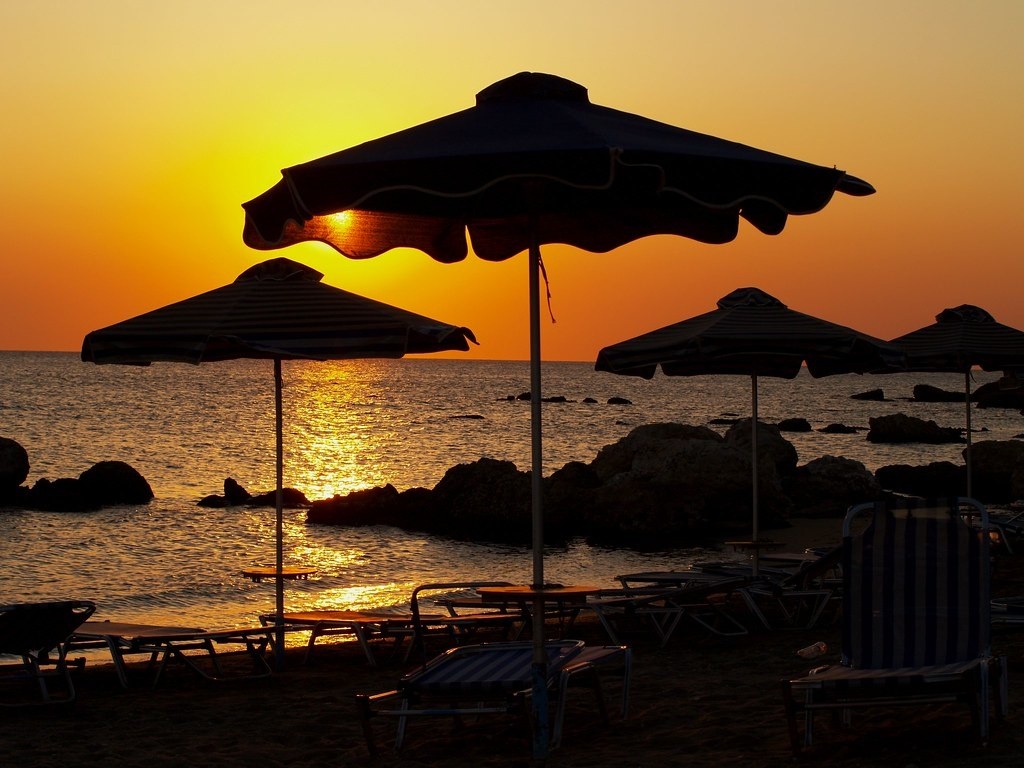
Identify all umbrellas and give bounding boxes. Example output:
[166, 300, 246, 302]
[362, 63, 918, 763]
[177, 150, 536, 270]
[79, 256, 481, 645]
[596, 287, 1024, 578]
[240, 71, 878, 665]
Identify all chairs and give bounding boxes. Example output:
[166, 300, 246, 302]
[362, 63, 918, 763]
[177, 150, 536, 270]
[0, 497, 1024, 762]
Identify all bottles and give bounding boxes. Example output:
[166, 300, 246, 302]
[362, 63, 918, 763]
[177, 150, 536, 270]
[796, 642, 830, 658]
